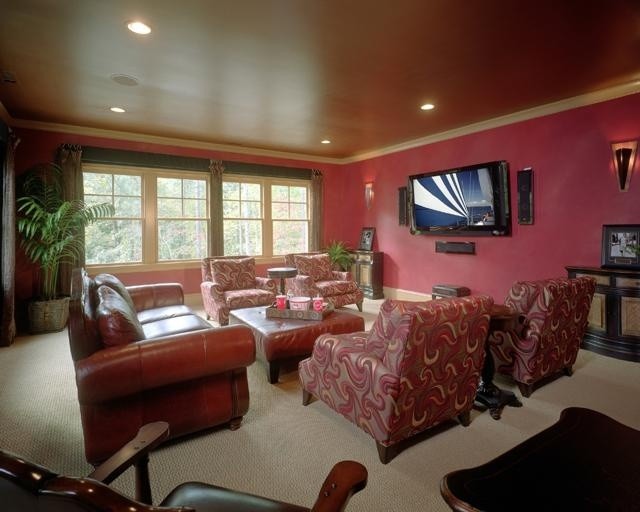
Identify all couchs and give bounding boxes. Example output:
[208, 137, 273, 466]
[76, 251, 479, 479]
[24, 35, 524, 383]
[67, 264, 257, 467]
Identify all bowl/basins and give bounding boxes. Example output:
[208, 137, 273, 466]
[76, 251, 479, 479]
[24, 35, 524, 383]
[289, 296, 312, 312]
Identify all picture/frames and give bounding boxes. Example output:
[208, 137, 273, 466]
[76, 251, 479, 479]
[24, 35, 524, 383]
[601, 221, 638, 271]
[357, 225, 376, 252]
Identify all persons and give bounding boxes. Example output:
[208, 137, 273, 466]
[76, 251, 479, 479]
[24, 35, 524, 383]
[619, 233, 628, 256]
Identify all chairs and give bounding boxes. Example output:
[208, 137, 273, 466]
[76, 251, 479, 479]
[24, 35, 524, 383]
[0, 417, 368, 511]
[200, 251, 364, 326]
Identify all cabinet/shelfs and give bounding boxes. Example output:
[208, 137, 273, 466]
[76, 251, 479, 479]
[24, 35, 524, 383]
[345, 252, 384, 299]
[565, 271, 640, 363]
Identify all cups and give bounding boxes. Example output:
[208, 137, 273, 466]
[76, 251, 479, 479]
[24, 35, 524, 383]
[275, 295, 287, 311]
[313, 297, 325, 312]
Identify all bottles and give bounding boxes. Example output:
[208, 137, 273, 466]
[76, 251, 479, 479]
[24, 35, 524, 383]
[286, 288, 295, 300]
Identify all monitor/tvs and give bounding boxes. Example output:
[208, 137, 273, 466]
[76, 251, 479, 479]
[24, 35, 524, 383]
[409, 160, 512, 238]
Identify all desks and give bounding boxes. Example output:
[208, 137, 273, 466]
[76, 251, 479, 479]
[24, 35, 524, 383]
[432, 404, 640, 512]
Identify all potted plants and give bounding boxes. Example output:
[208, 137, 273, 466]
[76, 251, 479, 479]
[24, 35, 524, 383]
[14, 155, 118, 334]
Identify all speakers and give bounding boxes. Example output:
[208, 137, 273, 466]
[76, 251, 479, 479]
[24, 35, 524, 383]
[516, 168, 534, 225]
[398, 187, 409, 225]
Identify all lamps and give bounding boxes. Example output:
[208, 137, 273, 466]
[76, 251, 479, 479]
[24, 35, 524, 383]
[609, 141, 638, 194]
